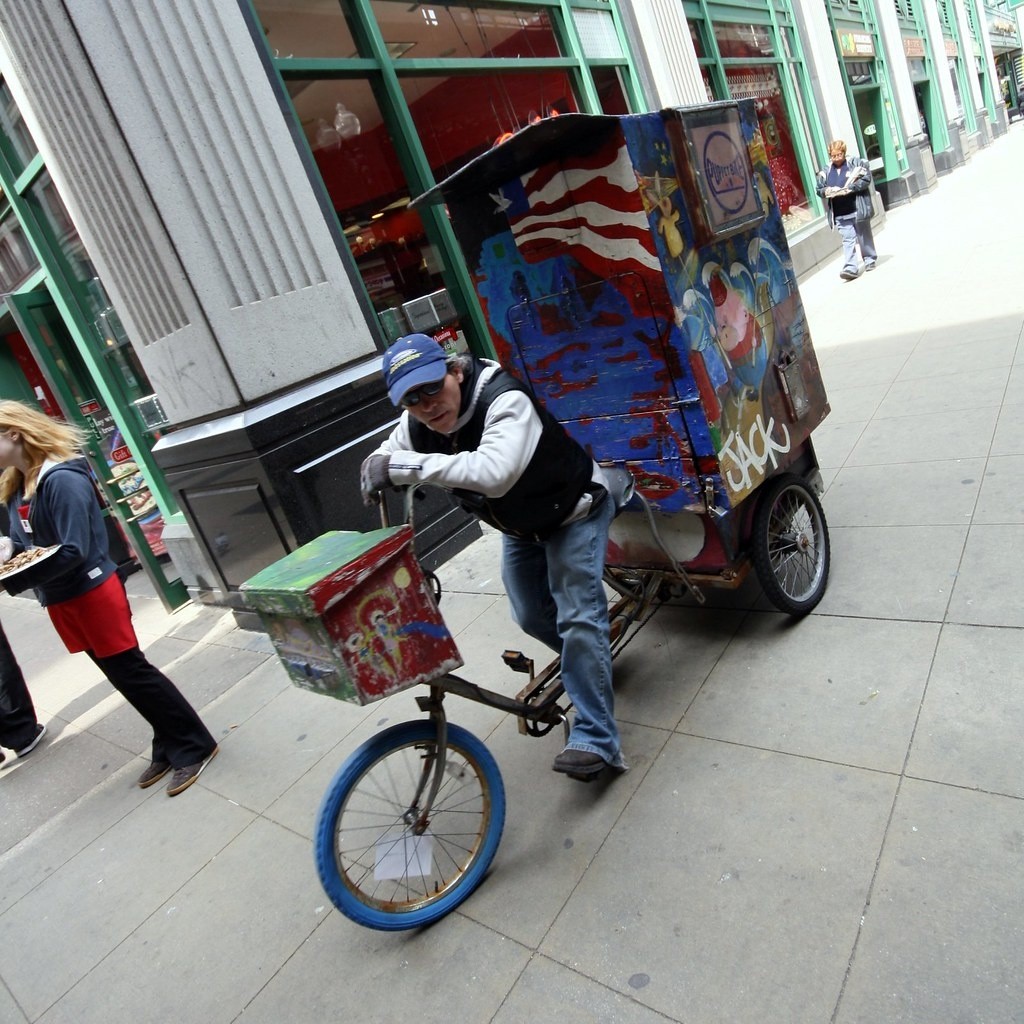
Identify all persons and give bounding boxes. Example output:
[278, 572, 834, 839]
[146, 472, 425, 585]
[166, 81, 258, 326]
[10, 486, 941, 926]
[816, 140, 876, 279]
[360, 333, 629, 782]
[0, 400, 220, 796]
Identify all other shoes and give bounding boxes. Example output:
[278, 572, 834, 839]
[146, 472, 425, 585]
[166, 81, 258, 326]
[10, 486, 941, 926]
[840, 270, 857, 278]
[866, 262, 877, 272]
[14, 723, 47, 756]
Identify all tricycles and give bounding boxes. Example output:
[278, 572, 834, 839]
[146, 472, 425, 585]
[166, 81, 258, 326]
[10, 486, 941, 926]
[237, 93, 832, 933]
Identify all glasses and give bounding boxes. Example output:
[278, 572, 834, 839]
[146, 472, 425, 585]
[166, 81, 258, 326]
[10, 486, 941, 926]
[402, 376, 445, 407]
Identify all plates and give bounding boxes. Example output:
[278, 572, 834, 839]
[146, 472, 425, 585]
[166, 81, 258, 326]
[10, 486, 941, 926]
[0, 545, 60, 580]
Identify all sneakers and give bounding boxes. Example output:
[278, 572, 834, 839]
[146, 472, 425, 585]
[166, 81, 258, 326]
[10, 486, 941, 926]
[138, 758, 174, 788]
[552, 748, 606, 775]
[165, 743, 219, 796]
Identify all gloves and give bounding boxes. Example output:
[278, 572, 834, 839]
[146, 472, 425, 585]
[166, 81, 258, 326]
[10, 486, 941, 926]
[359, 454, 394, 507]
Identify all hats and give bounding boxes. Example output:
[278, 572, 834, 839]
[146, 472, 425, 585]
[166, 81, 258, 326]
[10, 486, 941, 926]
[382, 333, 453, 406]
